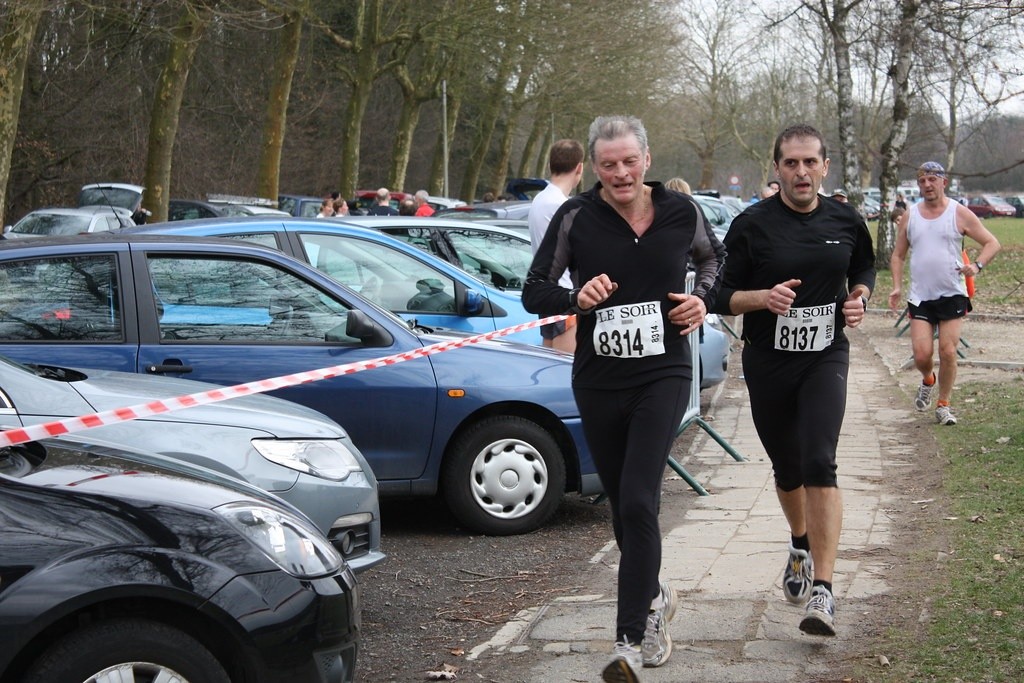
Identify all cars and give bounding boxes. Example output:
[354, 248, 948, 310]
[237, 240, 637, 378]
[0, 182, 745, 392]
[0, 234, 604, 539]
[857, 188, 922, 221]
[14, 214, 549, 348]
[999, 195, 1024, 219]
[0, 425, 364, 683]
[0, 347, 389, 577]
[964, 194, 1017, 220]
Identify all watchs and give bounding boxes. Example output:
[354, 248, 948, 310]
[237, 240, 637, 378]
[974, 261, 983, 272]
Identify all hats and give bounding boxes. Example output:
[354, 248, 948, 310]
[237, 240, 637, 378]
[829, 189, 847, 197]
[917, 161, 944, 179]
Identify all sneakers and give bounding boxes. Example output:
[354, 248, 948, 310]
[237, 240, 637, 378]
[936, 405, 956, 426]
[640, 583, 677, 667]
[602, 634, 642, 683]
[782, 542, 815, 604]
[914, 372, 937, 411]
[800, 584, 836, 638]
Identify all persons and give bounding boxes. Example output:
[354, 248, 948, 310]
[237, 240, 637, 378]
[526, 138, 585, 352]
[708, 124, 878, 630]
[750, 181, 780, 202]
[522, 113, 728, 683]
[889, 161, 1000, 424]
[688, 318, 692, 324]
[895, 193, 906, 210]
[830, 189, 848, 202]
[890, 207, 906, 225]
[316, 187, 436, 218]
[664, 177, 691, 195]
[483, 193, 495, 203]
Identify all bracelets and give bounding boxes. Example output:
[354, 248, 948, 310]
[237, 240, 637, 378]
[861, 296, 868, 312]
